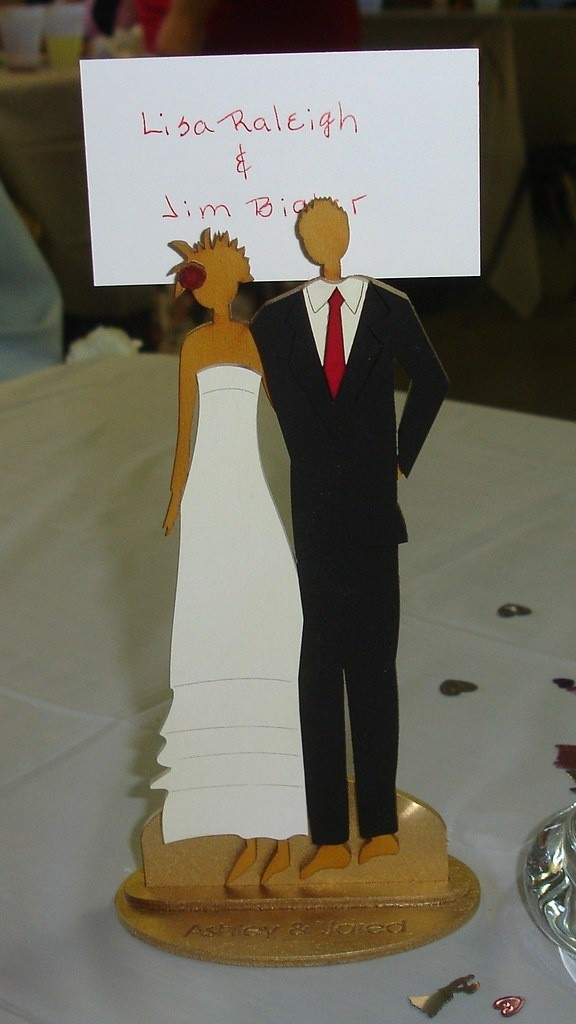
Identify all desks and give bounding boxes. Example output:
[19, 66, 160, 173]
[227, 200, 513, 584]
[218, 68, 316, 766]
[1, 356, 576, 1024]
[357, 8, 575, 318]
[1, 48, 153, 340]
